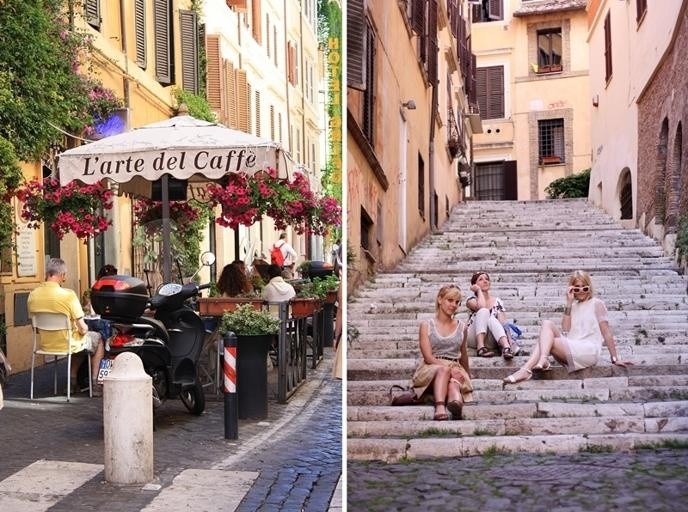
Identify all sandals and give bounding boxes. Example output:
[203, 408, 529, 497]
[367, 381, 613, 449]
[434, 401, 448, 420]
[531, 357, 553, 372]
[502, 367, 533, 384]
[503, 347, 514, 359]
[477, 347, 495, 357]
[448, 401, 463, 420]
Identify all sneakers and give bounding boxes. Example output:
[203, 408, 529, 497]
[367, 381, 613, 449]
[91, 384, 104, 397]
[63, 381, 80, 395]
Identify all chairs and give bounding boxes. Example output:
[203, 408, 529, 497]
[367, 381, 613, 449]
[27, 310, 95, 400]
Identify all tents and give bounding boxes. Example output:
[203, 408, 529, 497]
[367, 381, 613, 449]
[55, 115, 301, 285]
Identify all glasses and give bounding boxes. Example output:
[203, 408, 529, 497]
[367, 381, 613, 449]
[572, 286, 589, 293]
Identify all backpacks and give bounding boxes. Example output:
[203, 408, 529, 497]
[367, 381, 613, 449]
[271, 242, 288, 267]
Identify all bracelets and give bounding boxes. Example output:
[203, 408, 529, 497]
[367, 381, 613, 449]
[565, 307, 571, 315]
[612, 357, 617, 363]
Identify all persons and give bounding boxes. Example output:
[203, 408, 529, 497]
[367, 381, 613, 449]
[413, 284, 473, 420]
[260, 264, 295, 335]
[503, 270, 634, 385]
[462, 271, 515, 359]
[27, 259, 105, 397]
[271, 232, 298, 279]
[217, 264, 253, 298]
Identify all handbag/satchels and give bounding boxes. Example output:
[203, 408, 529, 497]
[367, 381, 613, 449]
[503, 321, 522, 356]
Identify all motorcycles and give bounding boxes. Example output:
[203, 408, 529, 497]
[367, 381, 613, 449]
[89, 250, 216, 429]
